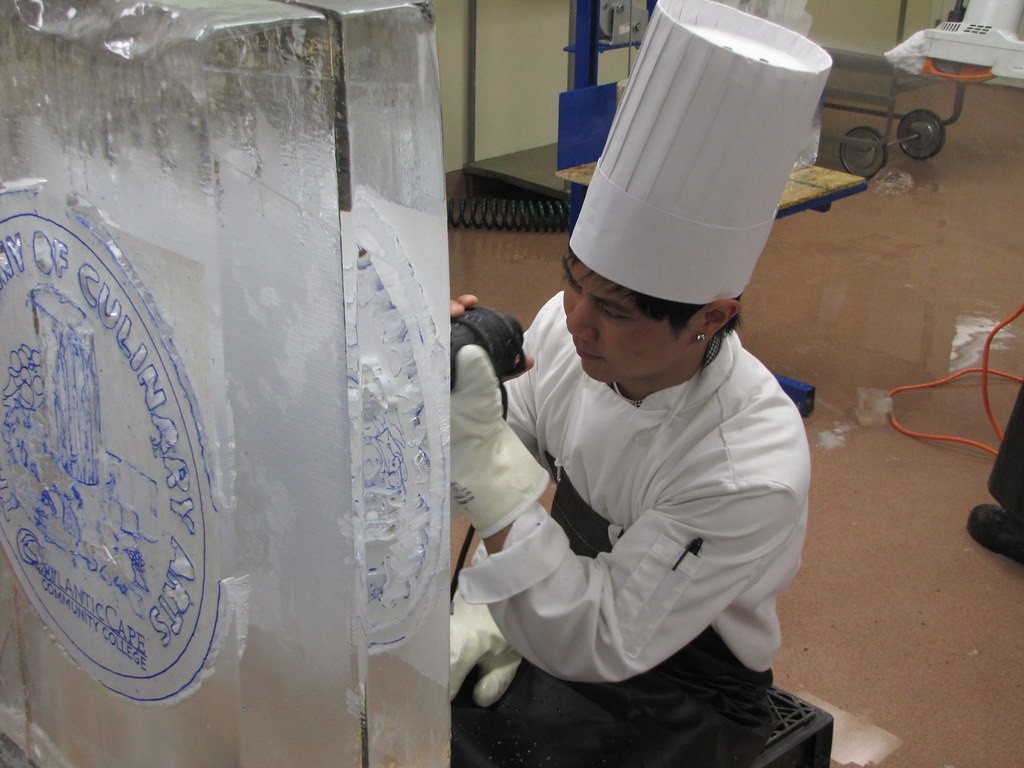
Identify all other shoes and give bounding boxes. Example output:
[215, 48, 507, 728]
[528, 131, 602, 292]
[966, 503, 1024, 564]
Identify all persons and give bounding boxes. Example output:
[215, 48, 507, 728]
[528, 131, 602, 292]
[967, 384, 1024, 565]
[450, 0, 833, 768]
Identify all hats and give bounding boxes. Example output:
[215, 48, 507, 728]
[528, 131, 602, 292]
[568, 0, 834, 306]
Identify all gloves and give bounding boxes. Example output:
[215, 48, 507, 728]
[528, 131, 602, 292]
[449, 343, 550, 539]
